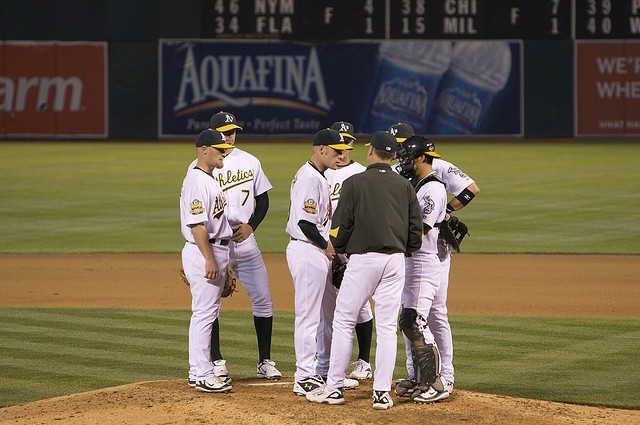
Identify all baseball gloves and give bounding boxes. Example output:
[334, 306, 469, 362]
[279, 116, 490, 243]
[332, 254, 347, 289]
[220, 265, 238, 298]
[440, 216, 468, 253]
[177, 267, 190, 286]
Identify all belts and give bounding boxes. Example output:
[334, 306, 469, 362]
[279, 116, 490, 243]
[209, 238, 229, 245]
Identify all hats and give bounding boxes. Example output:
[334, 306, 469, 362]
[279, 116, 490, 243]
[209, 110, 243, 131]
[365, 130, 396, 150]
[388, 121, 415, 142]
[330, 120, 356, 139]
[196, 129, 235, 148]
[313, 127, 353, 150]
[403, 135, 441, 157]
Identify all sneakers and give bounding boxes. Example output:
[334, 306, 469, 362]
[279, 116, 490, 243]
[343, 377, 359, 388]
[394, 381, 419, 395]
[412, 380, 448, 401]
[187, 378, 198, 386]
[350, 359, 373, 378]
[195, 375, 232, 391]
[213, 359, 228, 376]
[293, 377, 326, 395]
[372, 390, 393, 409]
[305, 385, 345, 403]
[441, 375, 453, 392]
[256, 359, 282, 379]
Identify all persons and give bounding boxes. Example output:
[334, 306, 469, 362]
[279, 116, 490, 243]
[186, 111, 282, 383]
[183, 128, 236, 394]
[306, 129, 421, 410]
[398, 138, 449, 405]
[389, 119, 480, 398]
[319, 121, 374, 381]
[288, 129, 358, 398]
